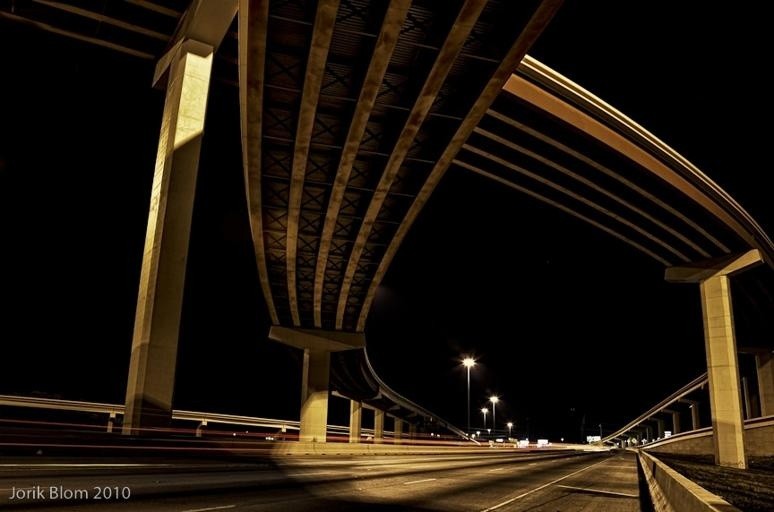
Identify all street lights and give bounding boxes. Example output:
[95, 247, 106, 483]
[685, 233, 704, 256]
[463, 357, 514, 439]
